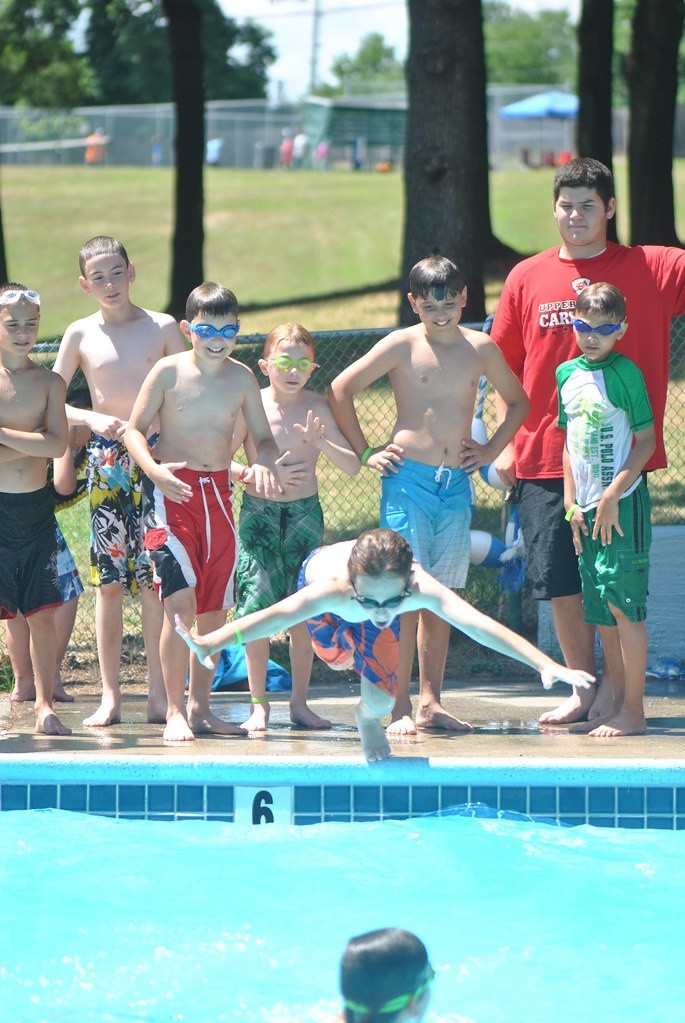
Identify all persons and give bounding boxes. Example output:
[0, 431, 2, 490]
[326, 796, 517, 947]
[124, 282, 284, 741]
[489, 158, 685, 724]
[328, 256, 530, 734]
[205, 130, 222, 167]
[84, 127, 105, 165]
[336, 927, 435, 1023]
[229, 322, 360, 731]
[0, 283, 95, 733]
[177, 528, 597, 763]
[555, 282, 656, 736]
[278, 125, 329, 169]
[53, 236, 186, 727]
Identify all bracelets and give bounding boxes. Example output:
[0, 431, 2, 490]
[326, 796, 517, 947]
[361, 447, 372, 465]
[225, 623, 242, 648]
[565, 504, 578, 521]
[239, 467, 249, 484]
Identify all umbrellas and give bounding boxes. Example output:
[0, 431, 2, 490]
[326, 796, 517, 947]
[499, 88, 580, 166]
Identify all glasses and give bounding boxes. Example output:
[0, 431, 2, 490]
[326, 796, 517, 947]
[570, 316, 628, 336]
[188, 322, 241, 340]
[267, 357, 320, 374]
[349, 578, 413, 610]
[0, 290, 41, 305]
